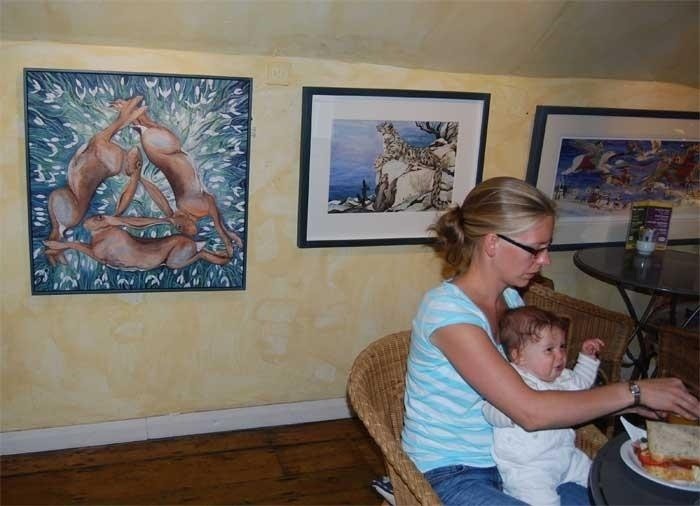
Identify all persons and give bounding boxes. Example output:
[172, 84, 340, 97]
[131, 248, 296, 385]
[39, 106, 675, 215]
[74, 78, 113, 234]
[401, 176, 700, 506]
[482, 308, 606, 506]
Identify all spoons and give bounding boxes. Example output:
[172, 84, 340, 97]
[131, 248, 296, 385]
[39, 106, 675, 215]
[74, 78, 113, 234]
[620, 416, 647, 441]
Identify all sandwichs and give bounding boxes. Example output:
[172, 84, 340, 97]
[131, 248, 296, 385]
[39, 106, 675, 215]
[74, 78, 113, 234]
[631, 420, 700, 481]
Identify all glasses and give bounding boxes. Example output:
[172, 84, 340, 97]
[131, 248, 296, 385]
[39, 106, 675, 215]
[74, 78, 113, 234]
[496, 232, 555, 260]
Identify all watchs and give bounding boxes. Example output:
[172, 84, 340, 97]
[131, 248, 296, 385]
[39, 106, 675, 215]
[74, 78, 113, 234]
[629, 380, 642, 405]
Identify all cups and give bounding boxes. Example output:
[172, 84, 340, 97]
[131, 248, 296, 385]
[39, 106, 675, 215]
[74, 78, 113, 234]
[636, 229, 658, 255]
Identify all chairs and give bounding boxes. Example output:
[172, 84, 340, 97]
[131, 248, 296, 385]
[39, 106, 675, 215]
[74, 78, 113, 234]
[654, 325, 700, 401]
[348, 325, 610, 503]
[520, 274, 639, 383]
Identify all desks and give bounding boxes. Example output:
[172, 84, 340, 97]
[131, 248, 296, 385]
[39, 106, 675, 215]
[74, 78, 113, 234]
[573, 248, 700, 380]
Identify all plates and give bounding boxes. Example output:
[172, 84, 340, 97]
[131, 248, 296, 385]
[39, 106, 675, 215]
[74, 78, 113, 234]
[619, 439, 700, 493]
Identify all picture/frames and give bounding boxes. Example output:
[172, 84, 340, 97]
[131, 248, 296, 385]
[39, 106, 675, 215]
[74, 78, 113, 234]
[298, 82, 493, 251]
[524, 104, 700, 251]
